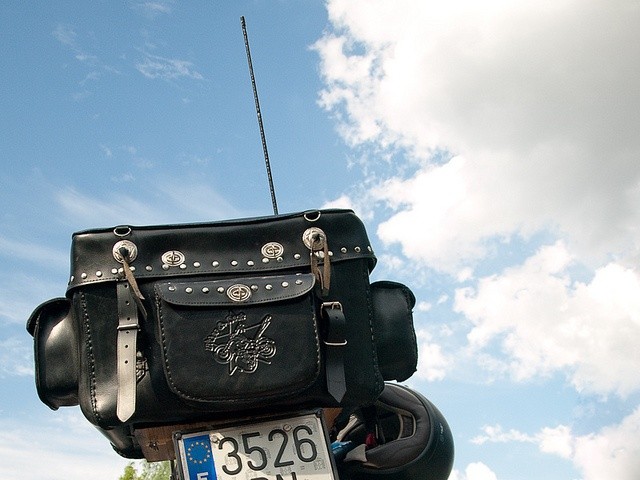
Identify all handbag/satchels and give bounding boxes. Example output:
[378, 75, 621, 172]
[25, 206, 420, 461]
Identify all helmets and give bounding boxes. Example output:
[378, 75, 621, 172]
[332, 382, 454, 480]
